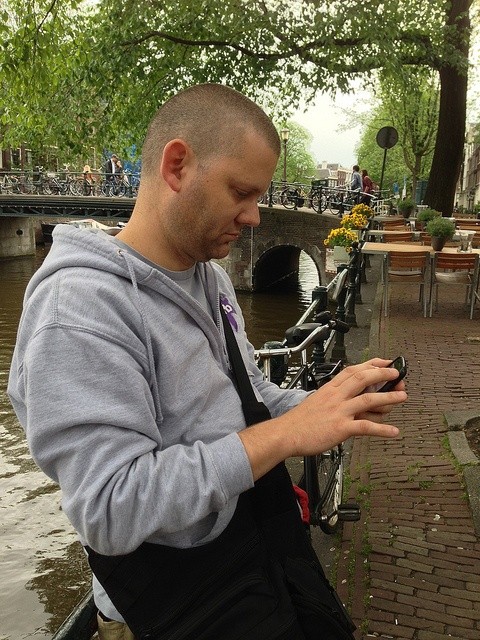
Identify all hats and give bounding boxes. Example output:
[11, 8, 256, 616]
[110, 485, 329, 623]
[113, 154, 119, 159]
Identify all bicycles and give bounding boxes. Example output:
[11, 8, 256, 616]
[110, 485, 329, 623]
[260, 174, 373, 216]
[2, 162, 140, 198]
[254, 311, 361, 536]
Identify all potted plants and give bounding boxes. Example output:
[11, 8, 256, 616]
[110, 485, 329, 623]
[423, 216, 457, 251]
[398, 197, 417, 218]
[415, 208, 441, 231]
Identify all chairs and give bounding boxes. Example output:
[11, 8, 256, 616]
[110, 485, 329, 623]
[429, 251, 480, 320]
[420, 236, 464, 248]
[380, 219, 407, 226]
[419, 232, 431, 238]
[380, 232, 415, 243]
[459, 225, 480, 247]
[383, 224, 411, 231]
[382, 250, 431, 319]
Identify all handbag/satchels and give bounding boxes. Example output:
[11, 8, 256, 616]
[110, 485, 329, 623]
[82, 399, 359, 638]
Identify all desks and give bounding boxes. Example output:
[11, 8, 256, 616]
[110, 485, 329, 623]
[360, 239, 480, 259]
[367, 229, 423, 238]
[374, 215, 418, 222]
[454, 218, 480, 223]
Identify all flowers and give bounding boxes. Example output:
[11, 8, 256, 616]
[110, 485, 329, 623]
[323, 227, 360, 253]
[340, 213, 369, 230]
[351, 203, 373, 214]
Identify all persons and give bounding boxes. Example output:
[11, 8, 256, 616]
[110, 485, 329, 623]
[7, 83, 408, 640]
[334, 165, 362, 202]
[392, 179, 400, 197]
[97, 154, 129, 186]
[360, 170, 373, 200]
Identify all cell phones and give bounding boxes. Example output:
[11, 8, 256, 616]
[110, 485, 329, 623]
[357, 355, 407, 395]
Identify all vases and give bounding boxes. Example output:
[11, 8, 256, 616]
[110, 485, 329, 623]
[351, 229, 362, 240]
[333, 245, 350, 261]
